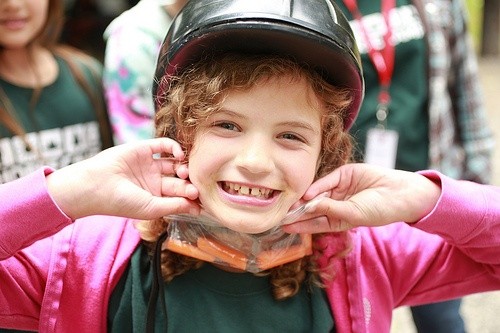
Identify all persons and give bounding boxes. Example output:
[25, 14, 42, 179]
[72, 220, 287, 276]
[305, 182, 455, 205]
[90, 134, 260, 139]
[0, 0, 107, 183]
[0, 0, 500, 332]
[334, 0, 495, 331]
[103, 0, 189, 145]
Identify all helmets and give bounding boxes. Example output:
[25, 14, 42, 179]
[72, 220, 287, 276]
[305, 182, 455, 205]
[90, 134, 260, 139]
[152, 0, 365, 133]
